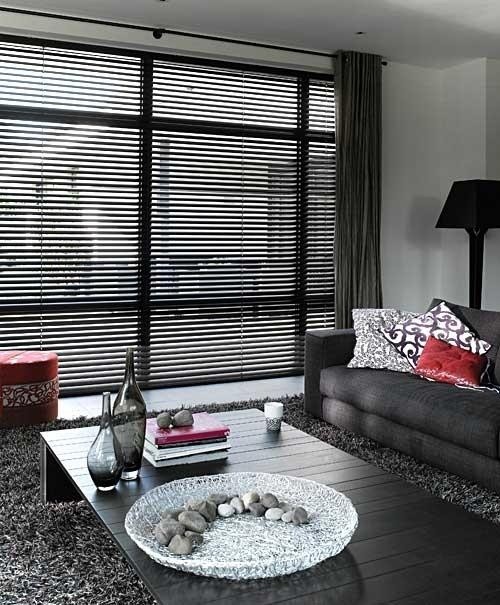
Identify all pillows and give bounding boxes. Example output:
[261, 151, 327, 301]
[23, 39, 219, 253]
[347, 309, 421, 374]
[376, 302, 492, 382]
[414, 335, 489, 389]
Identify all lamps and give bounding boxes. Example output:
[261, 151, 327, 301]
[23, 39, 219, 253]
[435, 179, 500, 309]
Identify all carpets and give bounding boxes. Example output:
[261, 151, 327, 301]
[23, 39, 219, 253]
[0, 394, 500, 605]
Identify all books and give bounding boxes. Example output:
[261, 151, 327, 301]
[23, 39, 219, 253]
[142, 410, 232, 468]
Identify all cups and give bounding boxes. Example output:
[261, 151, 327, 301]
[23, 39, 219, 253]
[264, 403, 284, 434]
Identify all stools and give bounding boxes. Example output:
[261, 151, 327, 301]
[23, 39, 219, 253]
[0, 351, 58, 427]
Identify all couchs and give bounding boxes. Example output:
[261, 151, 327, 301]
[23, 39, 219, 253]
[305, 297, 500, 495]
